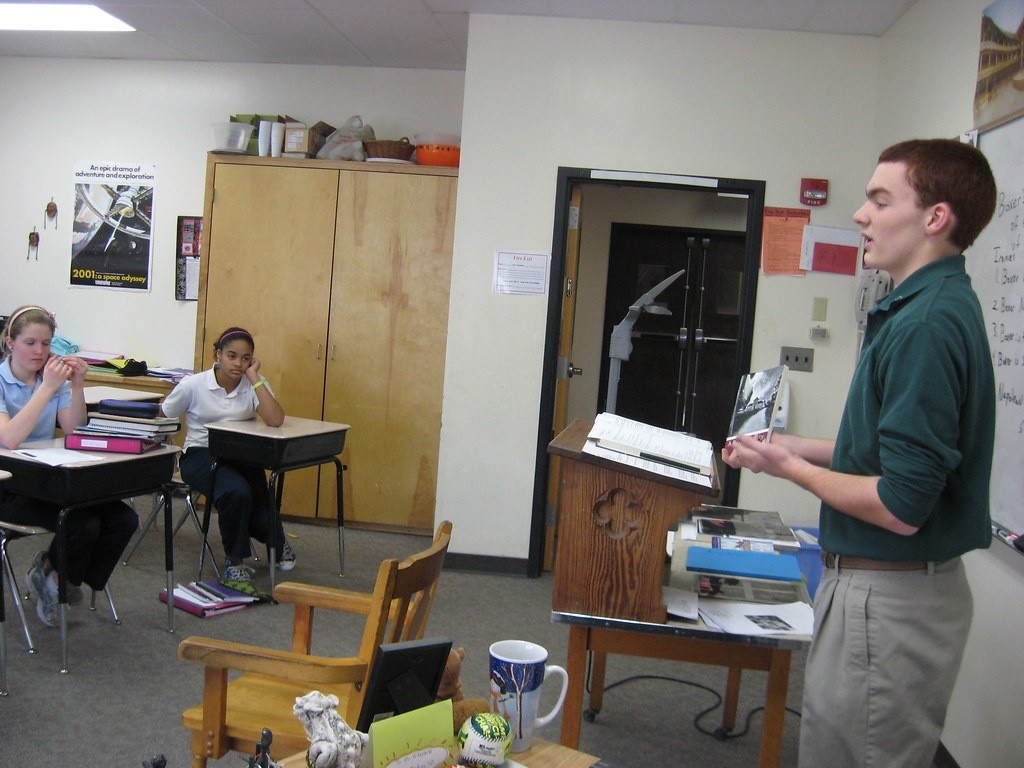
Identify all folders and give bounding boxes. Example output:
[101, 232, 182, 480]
[64, 434, 162, 454]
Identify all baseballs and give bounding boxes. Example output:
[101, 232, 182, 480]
[457, 713, 513, 768]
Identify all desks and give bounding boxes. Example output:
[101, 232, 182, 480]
[273, 744, 602, 768]
[80, 368, 190, 441]
[199, 414, 349, 585]
[2, 443, 181, 676]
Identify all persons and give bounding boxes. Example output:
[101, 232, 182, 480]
[160, 327, 296, 581]
[720, 140, 996, 768]
[0, 304, 139, 628]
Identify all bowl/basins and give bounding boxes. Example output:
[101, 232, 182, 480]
[211, 123, 256, 153]
[416, 144, 460, 167]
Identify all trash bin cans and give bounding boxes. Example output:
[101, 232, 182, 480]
[779, 527, 824, 602]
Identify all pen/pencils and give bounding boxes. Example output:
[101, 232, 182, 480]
[639, 452, 701, 472]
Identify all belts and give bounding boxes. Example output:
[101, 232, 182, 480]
[820, 546, 942, 571]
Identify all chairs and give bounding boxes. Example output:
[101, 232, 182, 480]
[179, 521, 454, 768]
[0, 469, 123, 674]
[123, 474, 223, 584]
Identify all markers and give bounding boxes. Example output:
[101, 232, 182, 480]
[997, 529, 1015, 544]
[1008, 531, 1018, 539]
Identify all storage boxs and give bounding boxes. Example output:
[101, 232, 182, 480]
[284, 114, 337, 152]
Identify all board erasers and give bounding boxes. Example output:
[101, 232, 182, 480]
[1013, 534, 1024, 554]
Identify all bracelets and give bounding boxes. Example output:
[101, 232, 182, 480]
[252, 381, 263, 390]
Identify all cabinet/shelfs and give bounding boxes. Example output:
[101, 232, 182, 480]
[191, 150, 458, 537]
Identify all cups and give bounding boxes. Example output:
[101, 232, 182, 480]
[258, 120, 271, 156]
[489, 640, 569, 753]
[271, 122, 285, 158]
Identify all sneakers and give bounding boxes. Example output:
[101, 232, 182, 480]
[33, 550, 88, 623]
[277, 540, 295, 571]
[26, 564, 62, 628]
[223, 556, 255, 583]
[118, 357, 147, 376]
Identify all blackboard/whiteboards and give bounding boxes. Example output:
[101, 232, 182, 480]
[950, 106, 1024, 558]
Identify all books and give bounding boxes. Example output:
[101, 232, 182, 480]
[724, 365, 790, 457]
[686, 545, 801, 588]
[355, 634, 452, 734]
[64, 411, 182, 454]
